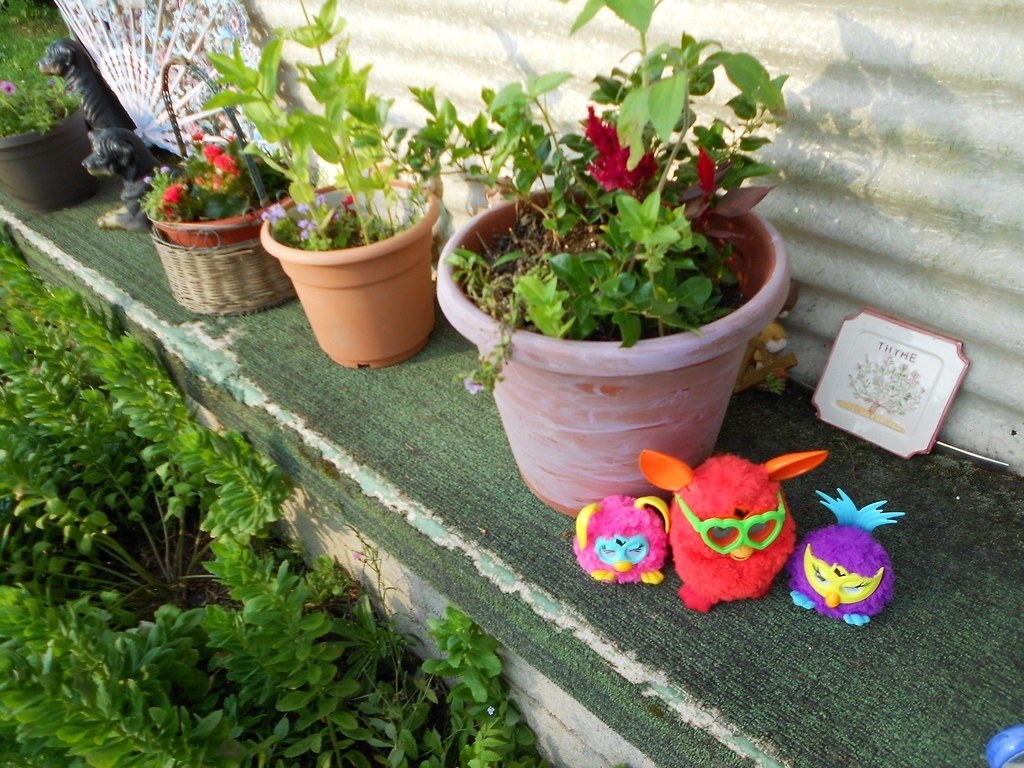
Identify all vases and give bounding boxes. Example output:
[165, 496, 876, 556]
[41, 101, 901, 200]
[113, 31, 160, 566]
[145, 200, 318, 320]
[419, 186, 798, 526]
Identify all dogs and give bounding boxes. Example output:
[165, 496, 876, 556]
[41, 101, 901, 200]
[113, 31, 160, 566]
[38, 39, 138, 135]
[81, 127, 169, 235]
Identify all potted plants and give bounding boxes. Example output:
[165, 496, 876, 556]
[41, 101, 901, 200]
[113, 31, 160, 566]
[199, 0, 450, 374]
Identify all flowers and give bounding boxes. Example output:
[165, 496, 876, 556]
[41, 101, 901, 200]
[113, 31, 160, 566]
[144, 116, 295, 217]
[409, 0, 803, 351]
